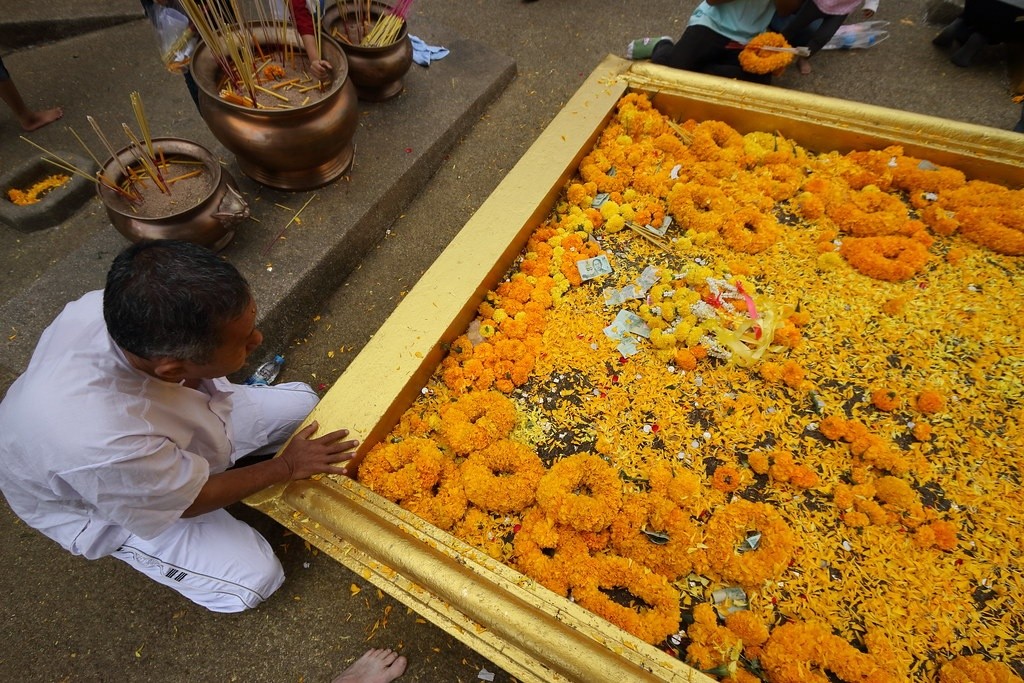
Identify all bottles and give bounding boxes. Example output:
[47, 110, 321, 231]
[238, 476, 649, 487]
[244, 355, 284, 386]
[831, 33, 878, 48]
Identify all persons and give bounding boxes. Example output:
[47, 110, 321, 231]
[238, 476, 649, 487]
[0, 55, 64, 131]
[140, 0, 333, 119]
[932, 0, 1024, 67]
[0, 238, 360, 613]
[626, 0, 805, 85]
[773, 0, 879, 78]
